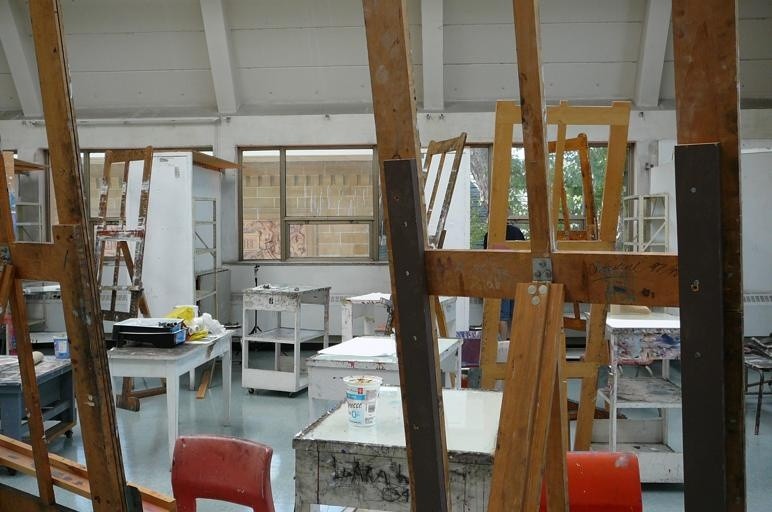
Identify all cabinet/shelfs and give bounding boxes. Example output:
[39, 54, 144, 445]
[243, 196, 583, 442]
[242, 285, 332, 393]
[1, 354, 77, 448]
[584, 310, 684, 484]
[305, 292, 464, 426]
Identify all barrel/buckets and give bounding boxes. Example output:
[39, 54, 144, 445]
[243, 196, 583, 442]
[53, 338, 70, 359]
[344, 375, 383, 427]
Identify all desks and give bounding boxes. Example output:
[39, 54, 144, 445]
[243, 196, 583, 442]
[107, 329, 234, 474]
[744, 336, 772, 435]
[292, 383, 504, 512]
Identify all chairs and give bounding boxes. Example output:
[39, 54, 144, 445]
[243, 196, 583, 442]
[172, 434, 276, 512]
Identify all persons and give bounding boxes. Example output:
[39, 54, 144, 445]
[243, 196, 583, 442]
[480, 225, 527, 340]
